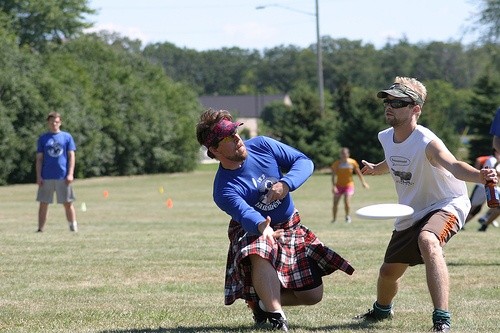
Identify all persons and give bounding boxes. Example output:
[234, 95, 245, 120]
[358, 77, 498, 333]
[197, 110, 355, 330]
[331, 147, 369, 224]
[459, 108, 500, 233]
[36, 112, 78, 232]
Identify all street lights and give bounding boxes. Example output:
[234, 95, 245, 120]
[255, 3, 327, 118]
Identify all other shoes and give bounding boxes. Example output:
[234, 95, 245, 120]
[429, 321, 450, 333]
[245, 298, 267, 324]
[345, 217, 351, 223]
[478, 218, 487, 225]
[331, 220, 336, 223]
[266, 312, 288, 331]
[364, 308, 390, 322]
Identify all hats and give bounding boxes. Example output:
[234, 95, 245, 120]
[377, 83, 424, 109]
[202, 118, 244, 148]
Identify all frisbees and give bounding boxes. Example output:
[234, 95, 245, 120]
[356, 204, 414, 220]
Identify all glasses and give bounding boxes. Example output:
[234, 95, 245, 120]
[383, 99, 418, 109]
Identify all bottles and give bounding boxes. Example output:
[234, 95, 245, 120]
[484, 165, 500, 208]
